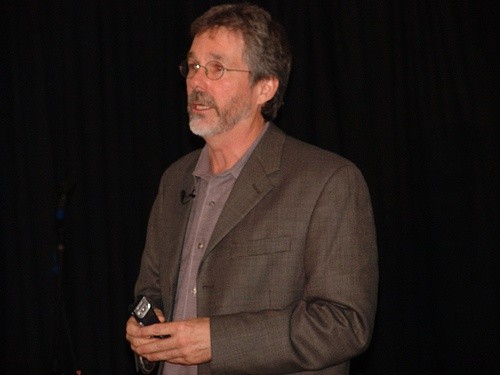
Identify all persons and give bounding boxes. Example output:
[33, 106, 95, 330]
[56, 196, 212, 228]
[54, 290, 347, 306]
[125, 1, 380, 375]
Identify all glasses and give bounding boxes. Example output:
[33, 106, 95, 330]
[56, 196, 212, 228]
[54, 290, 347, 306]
[179, 61, 252, 80]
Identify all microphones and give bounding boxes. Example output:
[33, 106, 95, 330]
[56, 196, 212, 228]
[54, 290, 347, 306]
[180, 190, 185, 204]
